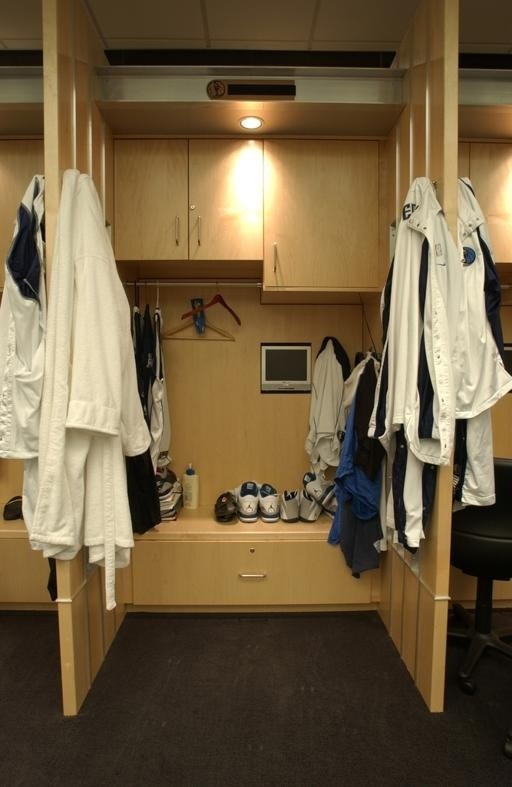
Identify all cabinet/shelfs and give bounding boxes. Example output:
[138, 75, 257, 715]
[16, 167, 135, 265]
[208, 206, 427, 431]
[131, 533, 372, 613]
[459, 137, 512, 264]
[263, 134, 388, 292]
[0, 532, 58, 611]
[112, 134, 263, 262]
[0, 135, 45, 293]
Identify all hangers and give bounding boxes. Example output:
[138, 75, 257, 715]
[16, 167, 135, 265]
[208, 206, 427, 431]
[160, 317, 235, 341]
[123, 278, 160, 323]
[181, 280, 241, 326]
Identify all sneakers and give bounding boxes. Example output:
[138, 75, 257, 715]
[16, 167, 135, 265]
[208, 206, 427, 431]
[215, 472, 337, 522]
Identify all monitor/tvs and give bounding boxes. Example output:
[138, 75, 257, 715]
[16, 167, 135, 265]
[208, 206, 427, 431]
[261, 346, 312, 392]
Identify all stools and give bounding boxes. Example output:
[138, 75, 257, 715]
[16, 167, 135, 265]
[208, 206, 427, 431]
[445, 471, 512, 695]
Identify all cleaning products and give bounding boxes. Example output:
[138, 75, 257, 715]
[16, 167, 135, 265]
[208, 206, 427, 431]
[181, 463, 200, 509]
